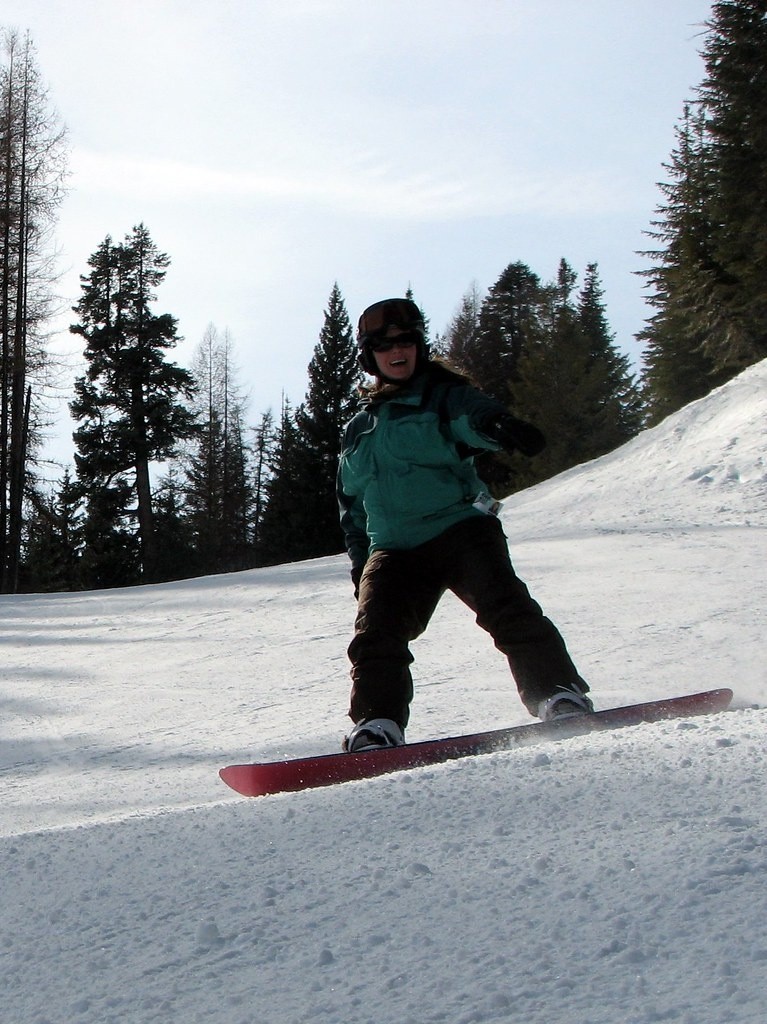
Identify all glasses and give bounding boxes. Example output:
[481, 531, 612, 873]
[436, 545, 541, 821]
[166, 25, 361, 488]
[369, 332, 417, 353]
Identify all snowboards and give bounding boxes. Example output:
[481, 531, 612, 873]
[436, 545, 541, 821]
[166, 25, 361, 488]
[216, 688, 737, 797]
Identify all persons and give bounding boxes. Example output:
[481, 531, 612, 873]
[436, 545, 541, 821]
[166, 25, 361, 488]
[334, 298, 596, 754]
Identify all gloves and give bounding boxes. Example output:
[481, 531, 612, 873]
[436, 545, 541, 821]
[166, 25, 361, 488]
[465, 400, 506, 433]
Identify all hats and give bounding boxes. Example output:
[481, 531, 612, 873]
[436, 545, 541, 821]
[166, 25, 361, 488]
[356, 299, 428, 374]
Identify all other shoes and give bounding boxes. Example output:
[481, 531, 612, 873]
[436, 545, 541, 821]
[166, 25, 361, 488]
[347, 727, 387, 751]
[547, 703, 586, 720]
